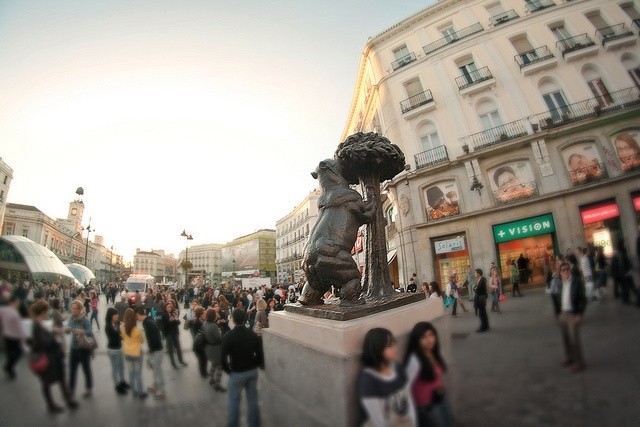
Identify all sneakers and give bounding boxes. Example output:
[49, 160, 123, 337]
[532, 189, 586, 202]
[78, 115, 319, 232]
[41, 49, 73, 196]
[133, 392, 138, 397]
[147, 386, 159, 393]
[138, 392, 147, 397]
[153, 391, 165, 400]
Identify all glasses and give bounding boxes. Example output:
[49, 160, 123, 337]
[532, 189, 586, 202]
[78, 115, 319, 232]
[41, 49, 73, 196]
[431, 197, 446, 210]
[617, 145, 633, 153]
[576, 158, 583, 171]
[499, 176, 514, 188]
[561, 268, 569, 272]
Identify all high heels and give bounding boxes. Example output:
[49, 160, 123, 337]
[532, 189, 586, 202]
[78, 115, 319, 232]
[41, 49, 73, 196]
[214, 383, 227, 393]
[209, 378, 214, 385]
[171, 362, 178, 370]
[180, 361, 189, 367]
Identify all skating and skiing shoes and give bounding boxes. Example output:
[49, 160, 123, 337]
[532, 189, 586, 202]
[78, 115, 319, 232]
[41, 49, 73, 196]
[82, 392, 93, 400]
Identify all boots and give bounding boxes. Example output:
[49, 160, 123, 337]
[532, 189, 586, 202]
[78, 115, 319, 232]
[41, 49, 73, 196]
[62, 386, 80, 409]
[490, 301, 496, 312]
[495, 302, 502, 314]
[42, 389, 65, 413]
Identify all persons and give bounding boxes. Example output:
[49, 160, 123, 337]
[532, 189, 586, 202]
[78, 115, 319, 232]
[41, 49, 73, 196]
[568, 153, 609, 186]
[101, 281, 124, 304]
[245, 290, 253, 307]
[220, 308, 266, 427]
[235, 285, 282, 298]
[168, 300, 179, 316]
[217, 295, 232, 320]
[115, 296, 129, 320]
[104, 307, 130, 395]
[169, 293, 180, 315]
[1, 275, 87, 309]
[424, 185, 460, 220]
[357, 327, 416, 427]
[153, 282, 184, 294]
[185, 299, 200, 324]
[612, 238, 639, 305]
[163, 303, 189, 369]
[135, 307, 170, 400]
[0, 295, 24, 379]
[558, 263, 587, 373]
[517, 253, 533, 283]
[211, 301, 231, 334]
[154, 293, 165, 314]
[614, 134, 640, 172]
[64, 300, 98, 399]
[134, 290, 141, 304]
[125, 289, 130, 302]
[88, 290, 101, 332]
[252, 299, 268, 345]
[545, 254, 562, 317]
[283, 281, 301, 292]
[412, 273, 416, 281]
[492, 166, 536, 204]
[240, 293, 249, 307]
[453, 273, 470, 312]
[50, 300, 69, 326]
[119, 308, 148, 398]
[28, 300, 81, 414]
[473, 268, 489, 333]
[510, 259, 524, 297]
[446, 276, 458, 315]
[189, 283, 224, 294]
[595, 246, 608, 297]
[565, 248, 577, 264]
[201, 293, 210, 308]
[246, 294, 260, 321]
[145, 288, 155, 315]
[203, 308, 226, 392]
[407, 278, 417, 292]
[429, 281, 442, 300]
[580, 248, 595, 299]
[266, 298, 274, 314]
[225, 287, 233, 314]
[489, 268, 502, 313]
[273, 295, 282, 311]
[190, 306, 210, 378]
[421, 282, 429, 294]
[405, 322, 456, 427]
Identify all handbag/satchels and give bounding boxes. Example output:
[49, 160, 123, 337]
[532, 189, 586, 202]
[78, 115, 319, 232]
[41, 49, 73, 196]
[74, 330, 95, 351]
[192, 324, 208, 352]
[30, 345, 48, 373]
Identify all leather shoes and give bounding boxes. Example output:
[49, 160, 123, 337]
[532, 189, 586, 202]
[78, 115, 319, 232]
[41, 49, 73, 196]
[477, 328, 482, 333]
[119, 379, 131, 388]
[569, 362, 583, 372]
[480, 328, 489, 332]
[562, 360, 575, 368]
[116, 383, 129, 395]
